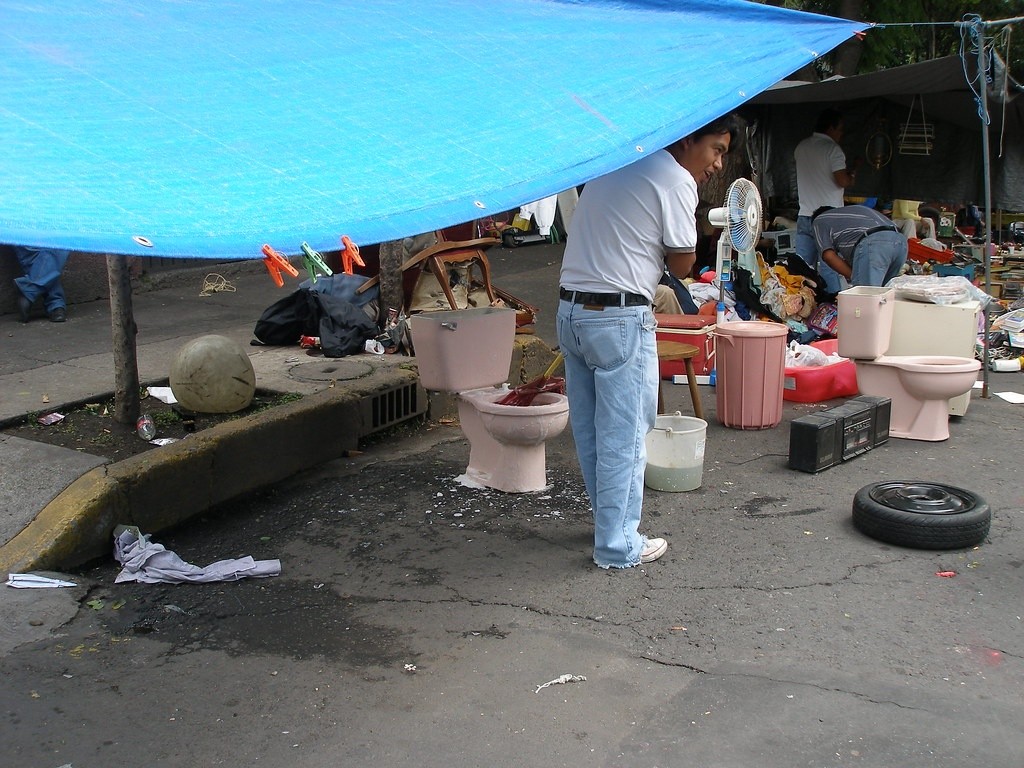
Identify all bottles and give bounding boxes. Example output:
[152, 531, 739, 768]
[137, 415, 156, 441]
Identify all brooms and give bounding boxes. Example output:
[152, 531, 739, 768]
[492, 350, 566, 407]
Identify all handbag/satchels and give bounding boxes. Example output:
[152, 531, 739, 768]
[249, 287, 330, 346]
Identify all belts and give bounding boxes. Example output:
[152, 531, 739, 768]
[850, 226, 901, 259]
[560, 286, 649, 307]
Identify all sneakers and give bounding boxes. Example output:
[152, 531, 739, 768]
[45, 307, 65, 321]
[641, 538, 667, 562]
[12, 279, 32, 322]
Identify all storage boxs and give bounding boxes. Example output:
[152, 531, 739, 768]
[783, 339, 858, 403]
[933, 263, 974, 282]
[937, 212, 956, 237]
[979, 286, 1002, 299]
[908, 238, 955, 266]
[655, 313, 717, 379]
[1004, 309, 1024, 328]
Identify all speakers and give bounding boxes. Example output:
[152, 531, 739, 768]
[853, 395, 892, 448]
[788, 415, 836, 474]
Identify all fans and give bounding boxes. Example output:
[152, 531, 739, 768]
[673, 177, 764, 388]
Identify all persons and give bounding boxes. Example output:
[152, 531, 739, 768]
[794, 112, 856, 293]
[12, 245, 71, 322]
[892, 199, 936, 239]
[811, 205, 908, 285]
[555, 113, 746, 569]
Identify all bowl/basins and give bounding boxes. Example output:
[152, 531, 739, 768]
[783, 338, 859, 403]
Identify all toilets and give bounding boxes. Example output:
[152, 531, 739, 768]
[409, 306, 570, 494]
[837, 285, 982, 442]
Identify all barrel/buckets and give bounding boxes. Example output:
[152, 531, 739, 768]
[644, 410, 709, 492]
[715, 321, 789, 430]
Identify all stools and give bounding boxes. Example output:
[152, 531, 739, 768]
[656, 340, 703, 419]
